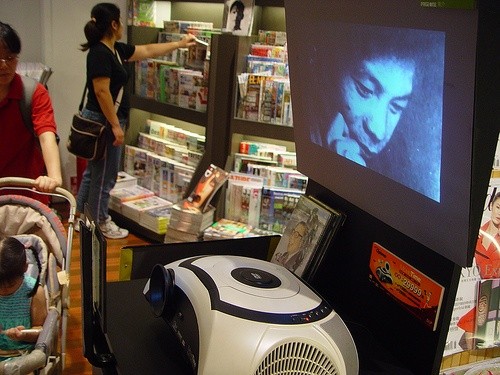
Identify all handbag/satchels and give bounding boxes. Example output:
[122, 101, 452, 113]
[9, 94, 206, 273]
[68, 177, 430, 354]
[67, 113, 108, 161]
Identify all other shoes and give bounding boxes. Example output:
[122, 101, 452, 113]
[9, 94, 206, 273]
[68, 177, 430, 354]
[75, 214, 129, 239]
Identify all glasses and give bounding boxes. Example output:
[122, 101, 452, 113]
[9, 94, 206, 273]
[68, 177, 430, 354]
[0, 56, 20, 64]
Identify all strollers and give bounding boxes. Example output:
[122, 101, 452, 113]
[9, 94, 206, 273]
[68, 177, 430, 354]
[0, 176, 78, 375]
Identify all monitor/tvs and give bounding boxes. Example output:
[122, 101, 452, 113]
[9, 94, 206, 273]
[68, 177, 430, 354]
[285, 0, 478, 267]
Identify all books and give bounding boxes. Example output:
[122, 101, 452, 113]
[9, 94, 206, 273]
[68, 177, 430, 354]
[127, 0, 171, 28]
[238, 30, 293, 124]
[106, 120, 205, 234]
[224, 0, 255, 36]
[128, 20, 220, 113]
[164, 142, 343, 283]
[443, 170, 500, 355]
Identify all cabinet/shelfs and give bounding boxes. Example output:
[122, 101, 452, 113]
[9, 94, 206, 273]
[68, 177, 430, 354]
[108, 25, 308, 246]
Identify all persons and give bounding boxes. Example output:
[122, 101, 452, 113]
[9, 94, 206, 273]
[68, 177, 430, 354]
[303, 26, 444, 197]
[0, 22, 62, 207]
[0, 237, 47, 375]
[189, 168, 220, 208]
[474, 192, 500, 279]
[230, 1, 245, 31]
[74, 3, 196, 239]
